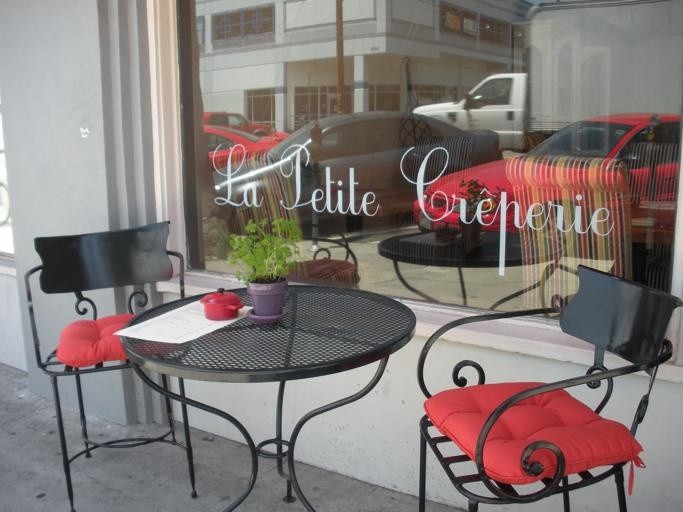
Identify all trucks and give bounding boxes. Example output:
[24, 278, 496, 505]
[413, 0, 682, 153]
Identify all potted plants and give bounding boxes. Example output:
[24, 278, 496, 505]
[450, 179, 497, 257]
[228, 216, 303, 323]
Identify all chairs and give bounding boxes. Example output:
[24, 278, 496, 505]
[418, 264, 682, 512]
[22, 219, 197, 512]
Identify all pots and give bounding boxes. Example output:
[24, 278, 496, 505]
[200, 289, 244, 321]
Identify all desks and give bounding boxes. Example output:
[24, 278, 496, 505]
[377, 227, 562, 311]
[118, 286, 415, 511]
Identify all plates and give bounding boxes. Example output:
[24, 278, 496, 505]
[248, 307, 291, 324]
